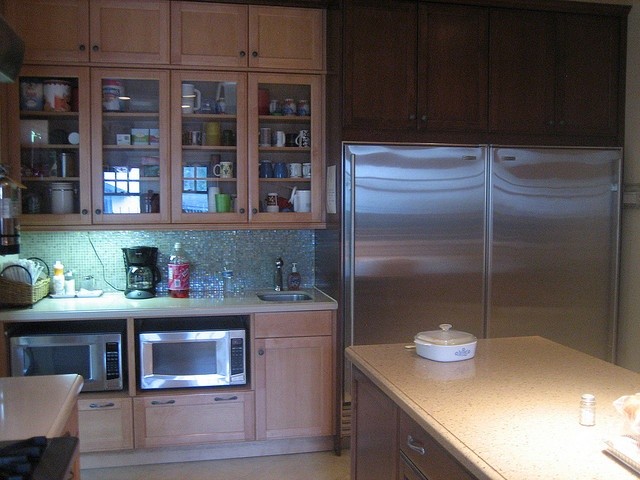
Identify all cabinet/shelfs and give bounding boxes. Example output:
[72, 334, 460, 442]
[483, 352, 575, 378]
[4, 0, 170, 68]
[349, 368, 478, 480]
[79, 398, 134, 454]
[57, 397, 81, 480]
[134, 390, 256, 451]
[171, 71, 321, 223]
[10, 70, 169, 226]
[172, 1, 325, 69]
[329, 2, 490, 138]
[491, 3, 620, 140]
[251, 311, 336, 441]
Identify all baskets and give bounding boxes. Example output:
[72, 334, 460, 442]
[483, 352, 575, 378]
[0, 258, 51, 306]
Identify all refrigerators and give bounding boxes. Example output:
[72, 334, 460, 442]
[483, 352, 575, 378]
[314, 140, 623, 452]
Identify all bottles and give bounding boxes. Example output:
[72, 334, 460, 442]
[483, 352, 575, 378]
[580, 393, 596, 426]
[167, 242, 191, 298]
[52, 260, 64, 296]
[64, 270, 75, 297]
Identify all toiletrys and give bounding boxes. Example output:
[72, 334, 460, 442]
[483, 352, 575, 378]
[288, 262, 301, 291]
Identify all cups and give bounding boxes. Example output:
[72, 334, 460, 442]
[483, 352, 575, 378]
[302, 162, 310, 178]
[288, 186, 311, 212]
[259, 159, 273, 177]
[295, 129, 311, 147]
[215, 193, 231, 212]
[289, 163, 302, 178]
[273, 162, 288, 178]
[266, 192, 280, 212]
[258, 127, 271, 147]
[297, 99, 309, 116]
[281, 98, 296, 115]
[213, 161, 234, 178]
[269, 99, 281, 116]
[286, 132, 298, 147]
[272, 130, 285, 147]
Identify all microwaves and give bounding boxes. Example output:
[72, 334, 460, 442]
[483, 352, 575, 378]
[140, 327, 246, 390]
[9, 332, 123, 392]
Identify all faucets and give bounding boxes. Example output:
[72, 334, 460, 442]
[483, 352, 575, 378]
[274, 258, 283, 291]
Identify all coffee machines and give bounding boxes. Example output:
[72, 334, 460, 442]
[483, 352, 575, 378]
[121, 246, 161, 299]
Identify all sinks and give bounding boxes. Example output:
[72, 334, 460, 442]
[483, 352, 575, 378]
[255, 293, 313, 301]
